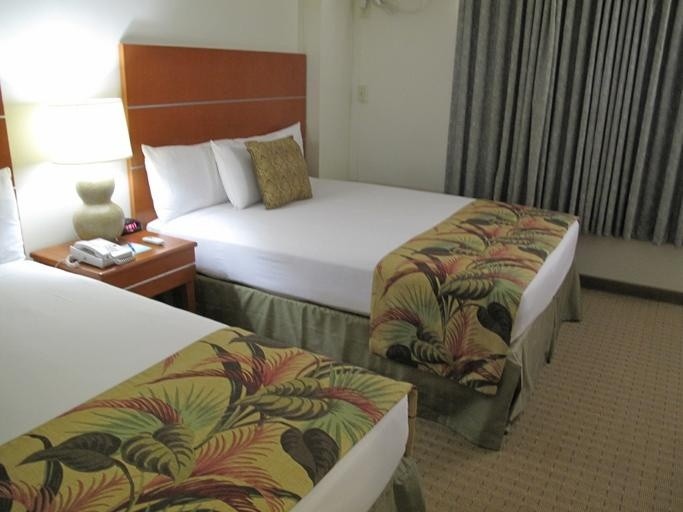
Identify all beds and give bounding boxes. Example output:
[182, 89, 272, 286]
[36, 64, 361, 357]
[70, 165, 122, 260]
[116, 40, 585, 453]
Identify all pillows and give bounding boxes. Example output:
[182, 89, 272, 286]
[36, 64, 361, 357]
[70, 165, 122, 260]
[246, 117, 306, 158]
[243, 134, 314, 210]
[139, 139, 229, 222]
[207, 134, 261, 210]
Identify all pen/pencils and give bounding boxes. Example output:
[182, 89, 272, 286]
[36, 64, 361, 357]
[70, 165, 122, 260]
[128, 243, 136, 253]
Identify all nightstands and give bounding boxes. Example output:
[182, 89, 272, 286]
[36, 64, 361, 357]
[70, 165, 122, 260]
[29, 230, 198, 313]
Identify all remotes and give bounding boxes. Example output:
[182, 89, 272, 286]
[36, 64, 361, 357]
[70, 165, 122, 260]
[142, 236, 163, 245]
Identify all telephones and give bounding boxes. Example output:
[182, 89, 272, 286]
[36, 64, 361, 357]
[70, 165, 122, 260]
[69, 237, 132, 269]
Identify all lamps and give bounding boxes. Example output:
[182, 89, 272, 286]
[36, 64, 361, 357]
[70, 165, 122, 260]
[63, 95, 133, 242]
